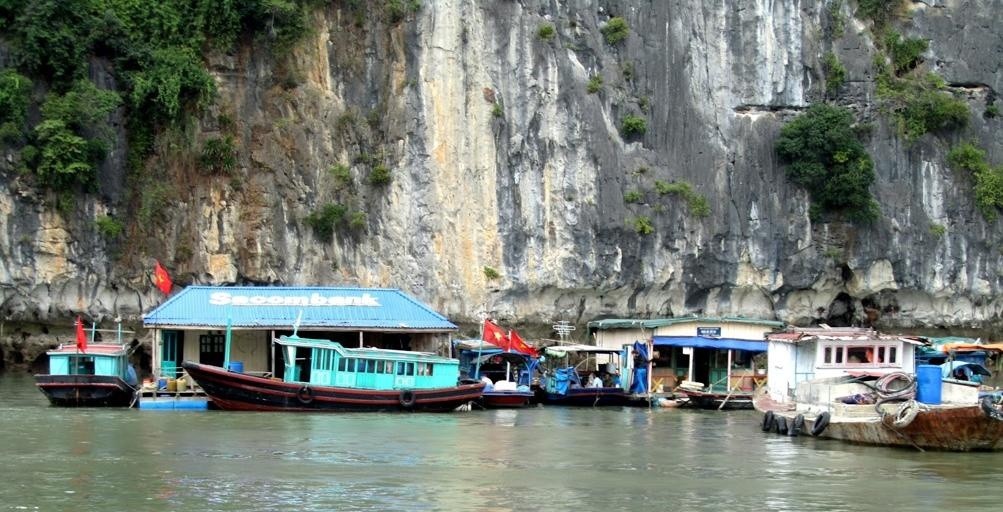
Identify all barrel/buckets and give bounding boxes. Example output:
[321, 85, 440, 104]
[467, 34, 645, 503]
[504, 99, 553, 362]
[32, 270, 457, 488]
[224, 361, 243, 375]
[126, 362, 137, 385]
[916, 365, 942, 404]
[634, 368, 647, 393]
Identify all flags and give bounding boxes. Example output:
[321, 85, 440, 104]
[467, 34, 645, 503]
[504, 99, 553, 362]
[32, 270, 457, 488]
[154, 259, 171, 296]
[507, 327, 538, 359]
[481, 318, 511, 353]
[76, 314, 88, 355]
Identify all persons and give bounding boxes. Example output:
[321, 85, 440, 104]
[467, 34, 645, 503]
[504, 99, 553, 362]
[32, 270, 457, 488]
[581, 366, 621, 389]
[629, 350, 647, 393]
[536, 355, 550, 375]
[954, 367, 968, 381]
[969, 369, 983, 384]
[652, 350, 664, 368]
[127, 362, 138, 389]
[126, 334, 150, 377]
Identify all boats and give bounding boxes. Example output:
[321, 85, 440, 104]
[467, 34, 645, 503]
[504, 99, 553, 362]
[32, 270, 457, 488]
[538, 345, 626, 405]
[181, 336, 485, 412]
[455, 339, 533, 406]
[752, 327, 1003, 454]
[33, 318, 145, 407]
